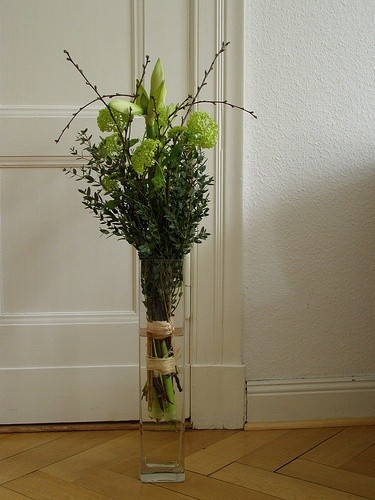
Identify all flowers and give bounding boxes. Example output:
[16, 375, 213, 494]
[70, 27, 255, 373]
[53, 40, 259, 427]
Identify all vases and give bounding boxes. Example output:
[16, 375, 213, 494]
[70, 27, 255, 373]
[136, 282, 193, 486]
